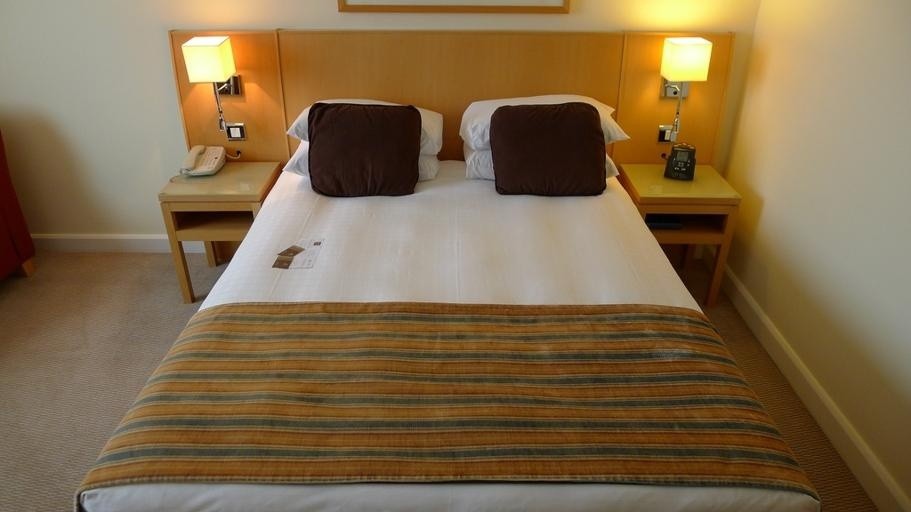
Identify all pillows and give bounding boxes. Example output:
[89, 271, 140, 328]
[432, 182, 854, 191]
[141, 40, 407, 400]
[310, 103, 421, 197]
[458, 94, 623, 148]
[285, 103, 439, 156]
[281, 140, 439, 183]
[487, 102, 601, 201]
[462, 143, 620, 184]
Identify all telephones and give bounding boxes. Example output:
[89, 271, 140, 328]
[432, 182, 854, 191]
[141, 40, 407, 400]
[183, 143, 226, 176]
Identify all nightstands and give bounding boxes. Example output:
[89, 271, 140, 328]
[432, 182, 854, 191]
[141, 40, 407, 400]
[614, 160, 743, 311]
[157, 160, 281, 306]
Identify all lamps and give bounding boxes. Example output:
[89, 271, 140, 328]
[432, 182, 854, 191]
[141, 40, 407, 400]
[659, 34, 713, 101]
[183, 35, 242, 100]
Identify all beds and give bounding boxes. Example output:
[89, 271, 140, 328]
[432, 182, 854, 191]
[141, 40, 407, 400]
[69, 137, 826, 512]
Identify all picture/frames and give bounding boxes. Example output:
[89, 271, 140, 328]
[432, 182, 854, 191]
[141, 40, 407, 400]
[337, 1, 571, 15]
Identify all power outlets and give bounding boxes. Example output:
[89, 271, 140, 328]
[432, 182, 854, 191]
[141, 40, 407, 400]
[658, 125, 674, 145]
[226, 125, 245, 143]
